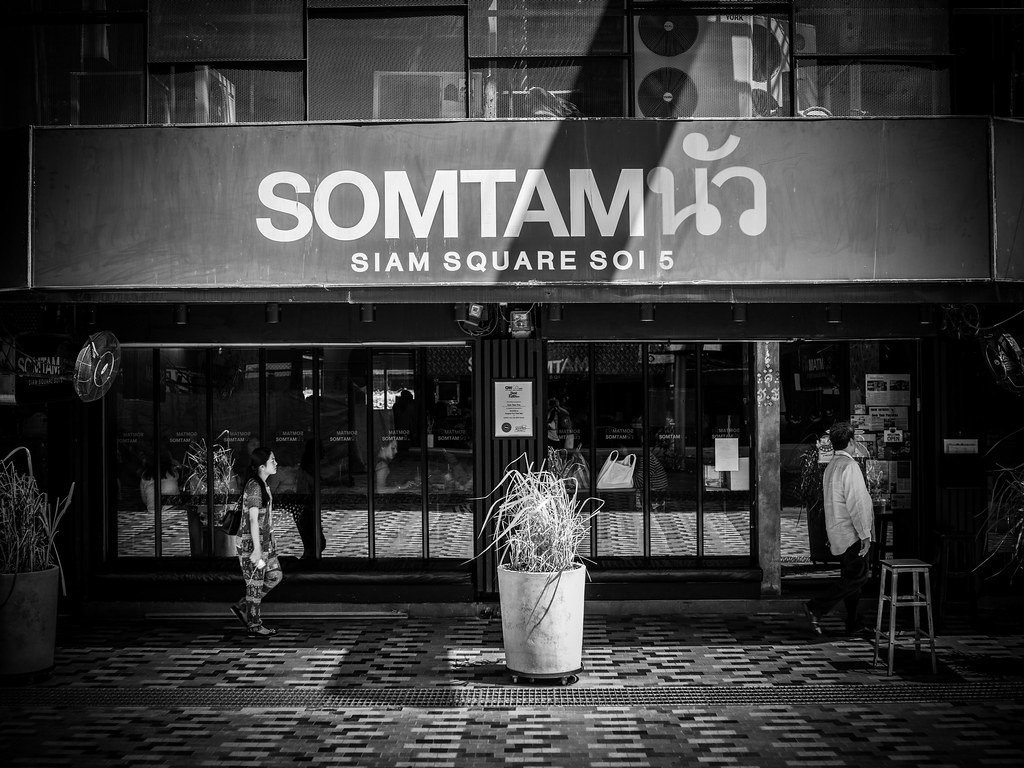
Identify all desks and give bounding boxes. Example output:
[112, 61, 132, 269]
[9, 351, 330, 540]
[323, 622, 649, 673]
[399, 481, 473, 553]
[705, 487, 730, 555]
[566, 488, 635, 510]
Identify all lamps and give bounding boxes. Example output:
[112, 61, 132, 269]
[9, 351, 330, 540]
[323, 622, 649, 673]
[88, 311, 96, 325]
[548, 303, 563, 321]
[359, 304, 376, 323]
[917, 304, 934, 325]
[173, 304, 190, 325]
[453, 303, 469, 321]
[265, 305, 281, 324]
[639, 304, 656, 325]
[826, 303, 843, 324]
[732, 303, 748, 323]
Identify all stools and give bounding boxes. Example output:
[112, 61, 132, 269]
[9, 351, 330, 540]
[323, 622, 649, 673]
[872, 559, 938, 677]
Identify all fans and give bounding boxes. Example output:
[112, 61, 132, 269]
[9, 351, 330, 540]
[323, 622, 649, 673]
[977, 325, 1024, 399]
[0, 331, 121, 402]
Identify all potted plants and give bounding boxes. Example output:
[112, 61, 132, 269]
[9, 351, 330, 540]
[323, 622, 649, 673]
[795, 449, 841, 564]
[459, 452, 604, 682]
[177, 430, 241, 558]
[0, 447, 75, 677]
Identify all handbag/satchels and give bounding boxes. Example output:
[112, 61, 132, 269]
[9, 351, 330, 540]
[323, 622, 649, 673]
[220, 494, 243, 535]
[281, 489, 297, 513]
[596, 450, 636, 490]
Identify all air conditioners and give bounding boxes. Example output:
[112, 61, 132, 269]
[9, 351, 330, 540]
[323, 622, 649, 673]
[753, 15, 818, 119]
[170, 65, 236, 124]
[623, 0, 753, 119]
[373, 70, 483, 118]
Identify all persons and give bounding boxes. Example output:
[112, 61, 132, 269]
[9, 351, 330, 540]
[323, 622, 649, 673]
[229, 448, 283, 638]
[546, 396, 669, 511]
[371, 389, 474, 494]
[801, 422, 877, 636]
[139, 435, 298, 511]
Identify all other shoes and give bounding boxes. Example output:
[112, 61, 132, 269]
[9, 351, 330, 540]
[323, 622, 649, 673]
[230, 606, 248, 628]
[803, 602, 822, 635]
[248, 629, 279, 639]
[847, 626, 875, 637]
